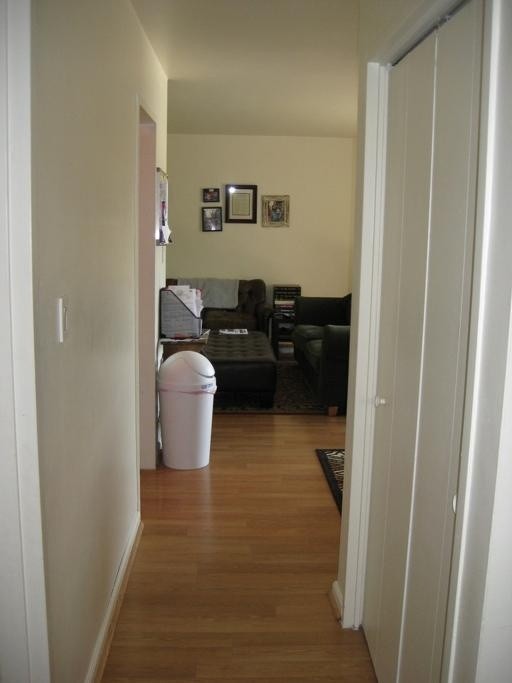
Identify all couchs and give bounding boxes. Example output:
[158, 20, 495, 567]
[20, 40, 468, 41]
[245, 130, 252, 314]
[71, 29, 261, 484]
[293, 294, 351, 417]
[166, 279, 267, 331]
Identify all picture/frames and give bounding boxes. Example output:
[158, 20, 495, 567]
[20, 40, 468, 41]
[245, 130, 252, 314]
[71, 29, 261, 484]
[261, 195, 290, 228]
[202, 188, 219, 202]
[225, 184, 256, 224]
[202, 207, 222, 231]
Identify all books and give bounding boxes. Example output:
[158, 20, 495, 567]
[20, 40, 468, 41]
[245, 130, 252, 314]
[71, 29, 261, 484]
[274, 300, 295, 319]
[220, 328, 249, 336]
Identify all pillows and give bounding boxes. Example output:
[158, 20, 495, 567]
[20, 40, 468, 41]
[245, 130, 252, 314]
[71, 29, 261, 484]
[314, 299, 332, 320]
[320, 300, 349, 319]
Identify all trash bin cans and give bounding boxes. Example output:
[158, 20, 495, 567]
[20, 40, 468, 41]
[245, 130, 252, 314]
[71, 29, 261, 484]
[156, 350, 217, 471]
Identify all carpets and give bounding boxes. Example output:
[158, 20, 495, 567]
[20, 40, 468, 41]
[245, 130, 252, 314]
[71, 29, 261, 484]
[315, 449, 345, 514]
[212, 351, 326, 416]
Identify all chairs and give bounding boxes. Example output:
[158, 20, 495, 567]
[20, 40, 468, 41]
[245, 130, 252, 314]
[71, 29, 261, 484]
[272, 286, 301, 361]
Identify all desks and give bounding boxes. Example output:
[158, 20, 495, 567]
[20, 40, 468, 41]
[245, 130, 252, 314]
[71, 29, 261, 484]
[161, 329, 211, 361]
